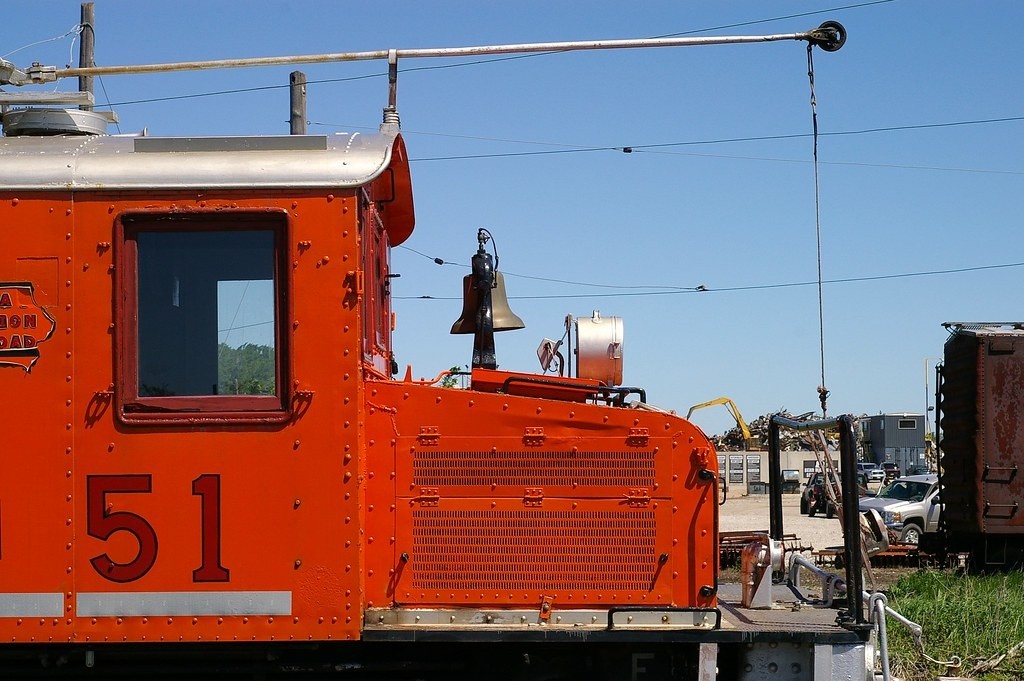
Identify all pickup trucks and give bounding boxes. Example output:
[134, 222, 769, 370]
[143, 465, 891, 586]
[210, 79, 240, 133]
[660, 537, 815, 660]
[801, 472, 841, 517]
[880, 462, 901, 479]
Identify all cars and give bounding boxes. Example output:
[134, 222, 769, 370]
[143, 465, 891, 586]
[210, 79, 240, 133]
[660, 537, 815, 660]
[906, 464, 928, 476]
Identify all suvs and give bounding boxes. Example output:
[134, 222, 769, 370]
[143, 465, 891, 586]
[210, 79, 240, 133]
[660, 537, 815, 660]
[857, 463, 885, 484]
[858, 473, 946, 549]
